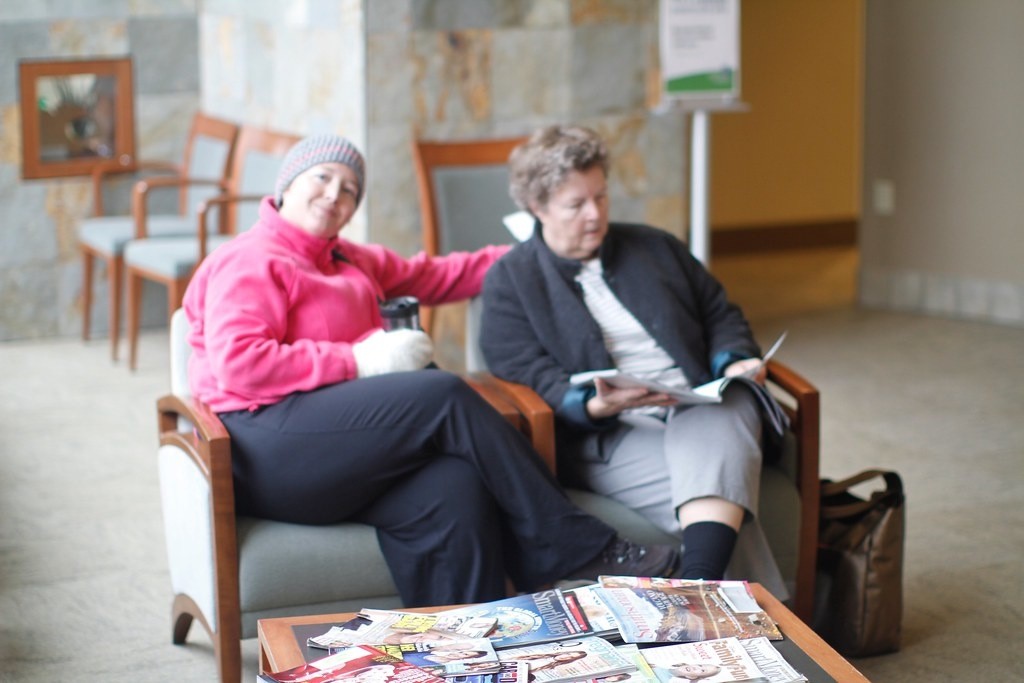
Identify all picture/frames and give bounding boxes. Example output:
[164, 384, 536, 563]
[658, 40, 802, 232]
[16, 56, 138, 180]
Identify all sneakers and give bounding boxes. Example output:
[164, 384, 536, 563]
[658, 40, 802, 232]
[553, 537, 677, 590]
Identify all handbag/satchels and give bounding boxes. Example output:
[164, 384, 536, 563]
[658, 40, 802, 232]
[817, 469, 906, 657]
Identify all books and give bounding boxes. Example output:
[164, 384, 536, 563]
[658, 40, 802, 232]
[570, 330, 791, 436]
[257, 575, 809, 683]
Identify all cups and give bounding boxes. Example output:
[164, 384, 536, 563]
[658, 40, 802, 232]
[379, 296, 420, 331]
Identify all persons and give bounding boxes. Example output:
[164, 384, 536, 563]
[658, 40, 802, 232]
[181, 136, 681, 608]
[478, 125, 790, 602]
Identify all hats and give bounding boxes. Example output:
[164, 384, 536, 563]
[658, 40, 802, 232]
[276, 134, 365, 211]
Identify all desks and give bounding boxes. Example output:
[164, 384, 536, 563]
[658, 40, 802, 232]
[258, 580, 871, 683]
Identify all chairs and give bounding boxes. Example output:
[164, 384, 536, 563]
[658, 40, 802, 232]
[124, 125, 304, 371]
[72, 112, 241, 363]
[152, 303, 525, 683]
[412, 135, 531, 341]
[465, 289, 821, 620]
